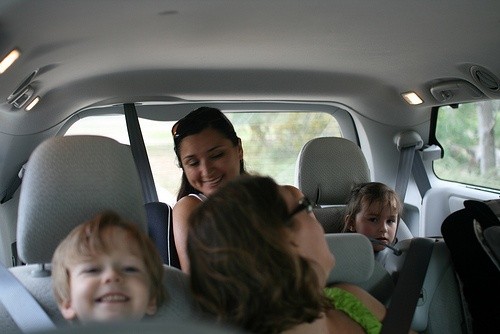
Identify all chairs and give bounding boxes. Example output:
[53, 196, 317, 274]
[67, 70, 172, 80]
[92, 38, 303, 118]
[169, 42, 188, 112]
[322, 234, 395, 306]
[0, 135, 191, 334]
[428, 199, 500, 334]
[297, 137, 413, 275]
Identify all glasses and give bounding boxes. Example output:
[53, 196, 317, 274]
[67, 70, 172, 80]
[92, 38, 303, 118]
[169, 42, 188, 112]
[284, 196, 314, 222]
[172, 109, 234, 139]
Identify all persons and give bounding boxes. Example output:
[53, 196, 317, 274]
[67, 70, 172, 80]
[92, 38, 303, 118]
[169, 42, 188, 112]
[343, 182, 402, 267]
[172, 106, 317, 274]
[52, 210, 171, 324]
[186, 175, 417, 334]
